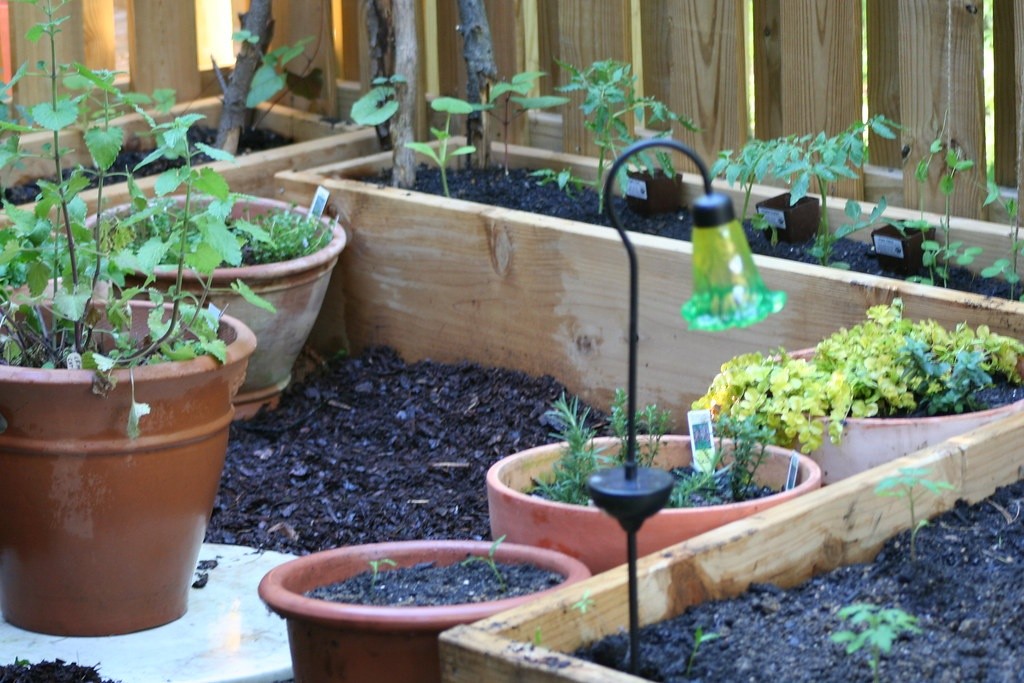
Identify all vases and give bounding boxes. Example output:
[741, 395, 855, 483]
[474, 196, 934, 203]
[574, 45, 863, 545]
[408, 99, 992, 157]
[871, 218, 937, 276]
[625, 169, 682, 214]
[756, 191, 820, 245]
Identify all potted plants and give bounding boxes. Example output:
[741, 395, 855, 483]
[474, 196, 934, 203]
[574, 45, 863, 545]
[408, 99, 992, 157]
[0, 1, 1023, 683]
